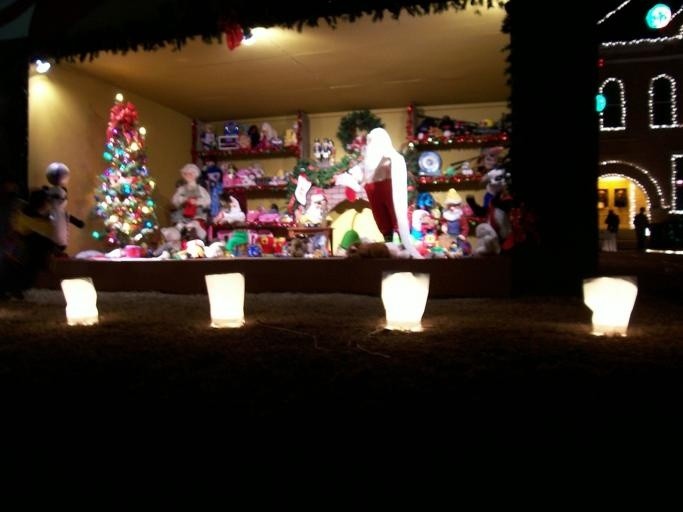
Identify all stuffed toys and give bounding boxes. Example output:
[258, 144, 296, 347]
[407, 149, 516, 262]
[167, 118, 333, 258]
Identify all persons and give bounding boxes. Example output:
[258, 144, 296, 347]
[603, 208, 620, 253]
[43, 160, 71, 256]
[633, 205, 650, 252]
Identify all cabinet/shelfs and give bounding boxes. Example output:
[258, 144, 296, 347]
[188, 110, 311, 240]
[405, 102, 512, 234]
